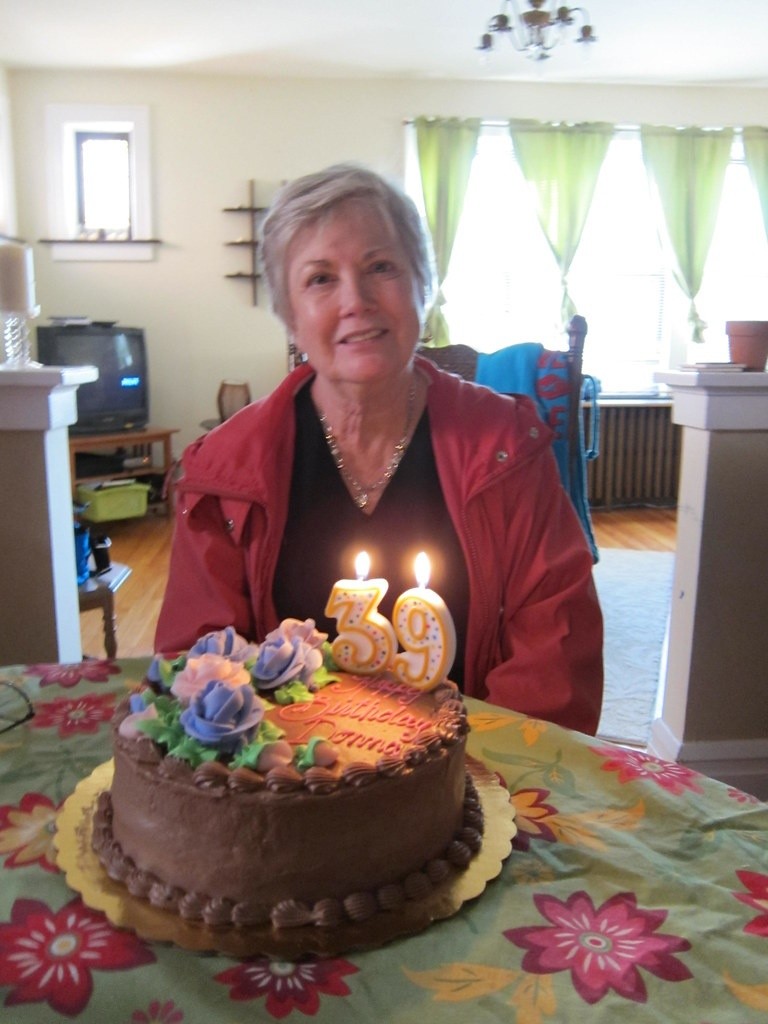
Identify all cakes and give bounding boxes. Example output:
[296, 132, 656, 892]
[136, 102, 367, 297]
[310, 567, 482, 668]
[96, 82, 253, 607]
[90, 577, 485, 927]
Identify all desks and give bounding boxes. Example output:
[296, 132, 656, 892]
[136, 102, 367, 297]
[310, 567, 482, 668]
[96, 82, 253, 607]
[0, 656, 768, 1024]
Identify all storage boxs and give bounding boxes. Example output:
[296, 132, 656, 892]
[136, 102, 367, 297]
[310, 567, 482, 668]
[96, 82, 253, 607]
[79, 480, 151, 522]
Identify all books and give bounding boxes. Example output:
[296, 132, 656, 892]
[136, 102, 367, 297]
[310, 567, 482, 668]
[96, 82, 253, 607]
[680, 361, 747, 373]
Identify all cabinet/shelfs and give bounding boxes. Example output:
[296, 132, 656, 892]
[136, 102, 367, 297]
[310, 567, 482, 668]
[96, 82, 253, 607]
[70, 427, 179, 524]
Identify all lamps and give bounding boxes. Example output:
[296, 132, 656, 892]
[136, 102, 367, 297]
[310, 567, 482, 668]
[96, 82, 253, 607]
[477, 0, 595, 60]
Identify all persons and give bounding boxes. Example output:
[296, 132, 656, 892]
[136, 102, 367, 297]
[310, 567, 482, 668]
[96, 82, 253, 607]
[152, 163, 604, 738]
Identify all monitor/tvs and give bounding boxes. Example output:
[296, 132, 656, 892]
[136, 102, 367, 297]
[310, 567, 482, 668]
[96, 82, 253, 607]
[37, 325, 149, 432]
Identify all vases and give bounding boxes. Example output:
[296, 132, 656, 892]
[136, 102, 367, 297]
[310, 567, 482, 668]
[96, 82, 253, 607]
[725, 321, 768, 371]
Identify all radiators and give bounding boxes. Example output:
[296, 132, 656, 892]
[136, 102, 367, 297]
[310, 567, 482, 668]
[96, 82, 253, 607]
[582, 400, 681, 508]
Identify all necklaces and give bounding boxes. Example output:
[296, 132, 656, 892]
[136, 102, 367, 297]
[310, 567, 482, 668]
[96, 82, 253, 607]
[307, 367, 418, 511]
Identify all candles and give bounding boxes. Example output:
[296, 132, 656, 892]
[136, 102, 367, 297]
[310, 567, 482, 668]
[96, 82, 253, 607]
[390, 585, 457, 691]
[325, 579, 398, 676]
